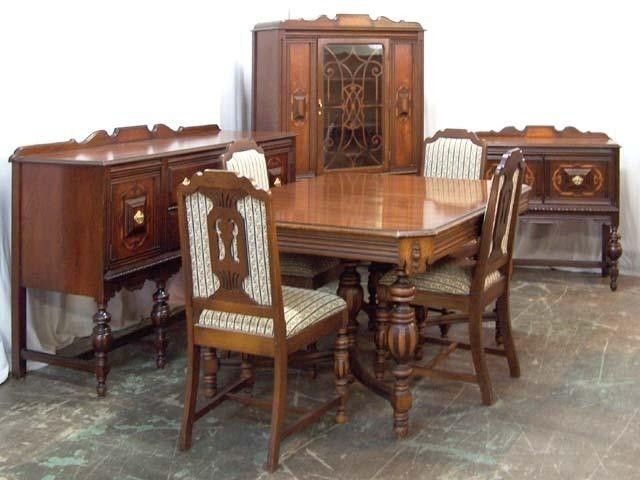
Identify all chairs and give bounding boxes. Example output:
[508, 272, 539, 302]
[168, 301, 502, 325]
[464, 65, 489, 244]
[175, 169, 349, 473]
[419, 128, 488, 180]
[374, 148, 526, 407]
[220, 138, 341, 379]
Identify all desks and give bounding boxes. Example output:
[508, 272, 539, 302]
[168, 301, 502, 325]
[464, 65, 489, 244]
[203, 172, 532, 440]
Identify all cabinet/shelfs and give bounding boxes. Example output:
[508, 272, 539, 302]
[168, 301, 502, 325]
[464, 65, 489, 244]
[8, 124, 298, 397]
[252, 14, 424, 176]
[424, 125, 621, 293]
